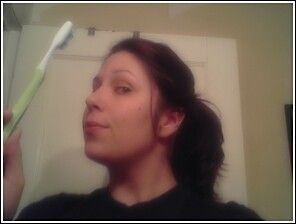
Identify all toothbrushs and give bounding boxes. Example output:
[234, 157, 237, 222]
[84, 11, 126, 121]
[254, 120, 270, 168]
[2, 20, 77, 145]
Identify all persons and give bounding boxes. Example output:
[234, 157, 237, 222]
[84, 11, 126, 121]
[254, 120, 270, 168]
[3, 38, 265, 220]
